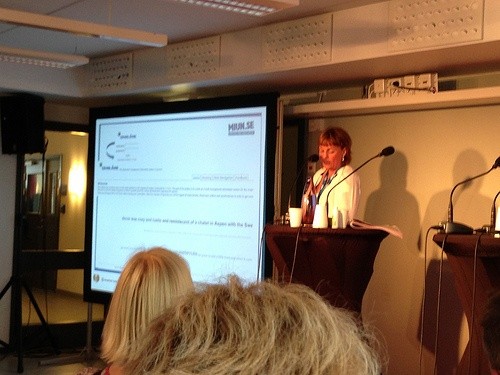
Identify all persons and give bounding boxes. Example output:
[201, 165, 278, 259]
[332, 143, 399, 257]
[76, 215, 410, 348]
[95, 247, 193, 375]
[128, 274, 385, 375]
[282, 125, 360, 227]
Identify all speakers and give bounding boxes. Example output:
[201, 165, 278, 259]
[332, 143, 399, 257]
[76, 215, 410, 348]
[0, 94, 45, 153]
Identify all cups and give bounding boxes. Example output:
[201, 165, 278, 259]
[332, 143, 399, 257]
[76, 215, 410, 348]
[313, 204, 328, 229]
[289, 207, 303, 228]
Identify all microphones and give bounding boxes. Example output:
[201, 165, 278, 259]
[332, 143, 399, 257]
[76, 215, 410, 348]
[437, 157, 500, 234]
[283, 154, 319, 224]
[321, 147, 395, 228]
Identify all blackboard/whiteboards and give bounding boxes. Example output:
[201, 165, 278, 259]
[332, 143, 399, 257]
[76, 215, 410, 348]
[0, 146, 26, 348]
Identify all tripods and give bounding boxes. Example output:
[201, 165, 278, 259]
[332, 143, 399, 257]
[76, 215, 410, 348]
[0, 154, 61, 374]
